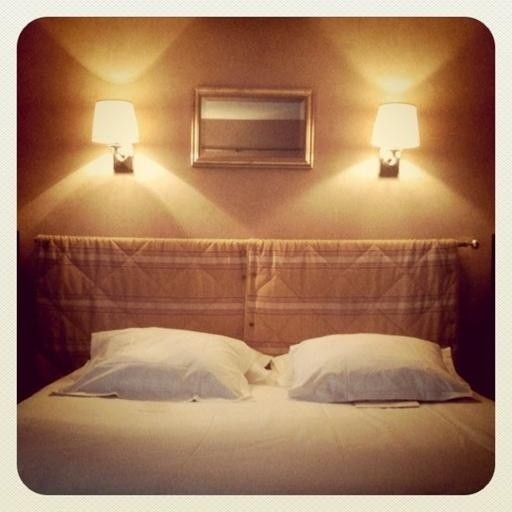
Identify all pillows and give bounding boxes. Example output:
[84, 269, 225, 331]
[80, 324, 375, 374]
[72, 330, 266, 403]
[274, 336, 475, 406]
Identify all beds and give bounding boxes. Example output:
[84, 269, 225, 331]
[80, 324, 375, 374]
[17, 234, 495, 495]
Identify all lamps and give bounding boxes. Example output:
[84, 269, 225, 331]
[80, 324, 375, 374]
[370, 102, 422, 180]
[89, 98, 141, 176]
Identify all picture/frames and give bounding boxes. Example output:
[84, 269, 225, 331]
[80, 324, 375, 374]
[190, 83, 318, 171]
